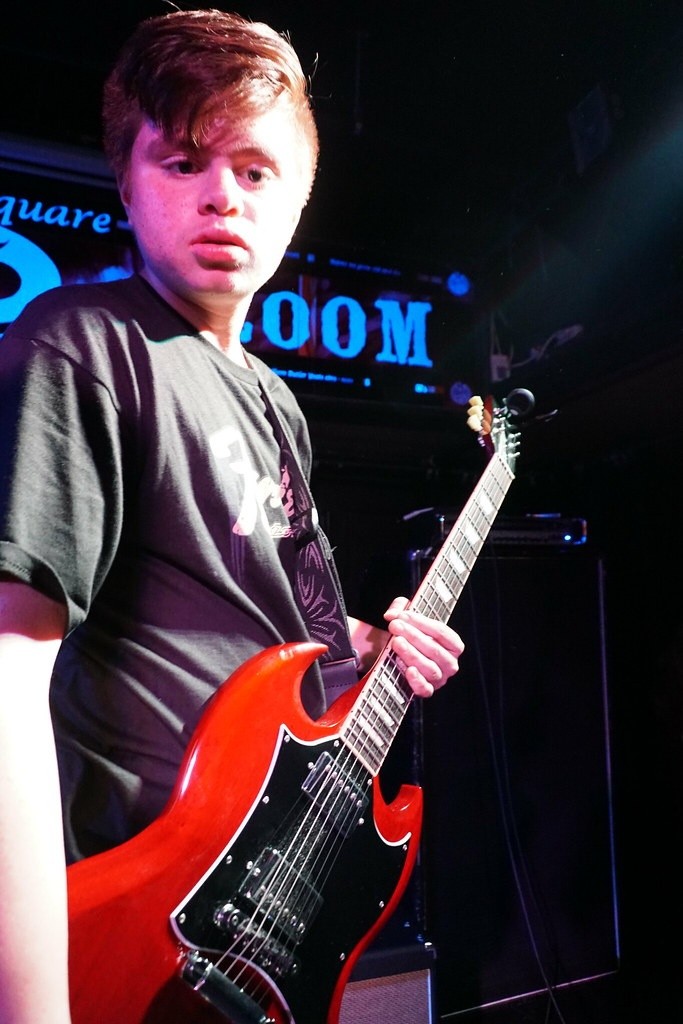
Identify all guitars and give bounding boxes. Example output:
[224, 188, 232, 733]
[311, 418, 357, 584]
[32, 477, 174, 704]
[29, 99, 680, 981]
[67, 388, 535, 1024]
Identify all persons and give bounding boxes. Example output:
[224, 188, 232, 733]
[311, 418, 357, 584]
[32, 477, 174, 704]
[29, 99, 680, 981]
[0, 13, 467, 1024]
